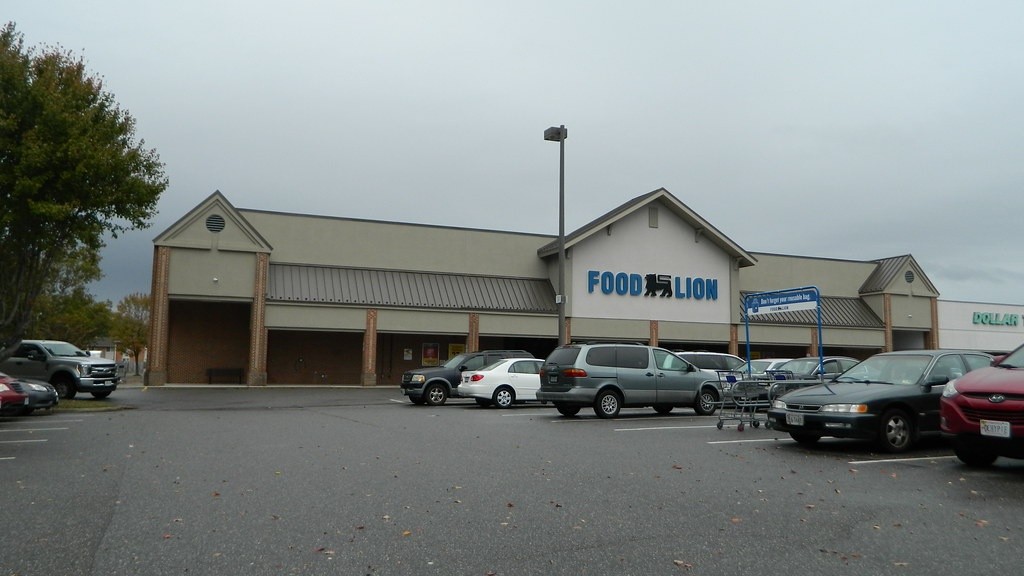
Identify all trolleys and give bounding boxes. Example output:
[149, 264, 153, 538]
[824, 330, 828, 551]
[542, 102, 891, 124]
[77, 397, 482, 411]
[714, 370, 760, 431]
[765, 369, 817, 429]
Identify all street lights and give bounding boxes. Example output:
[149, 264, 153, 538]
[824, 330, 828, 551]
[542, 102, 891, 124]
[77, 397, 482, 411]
[544, 125, 567, 345]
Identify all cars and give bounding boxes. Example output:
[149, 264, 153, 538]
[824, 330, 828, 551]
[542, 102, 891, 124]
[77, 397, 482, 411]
[0, 372, 58, 413]
[768, 350, 994, 454]
[457, 358, 544, 408]
[940, 343, 1024, 466]
[664, 349, 862, 406]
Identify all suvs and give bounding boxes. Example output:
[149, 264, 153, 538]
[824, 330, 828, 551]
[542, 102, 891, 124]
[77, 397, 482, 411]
[401, 348, 534, 407]
[537, 340, 727, 416]
[3, 339, 119, 400]
